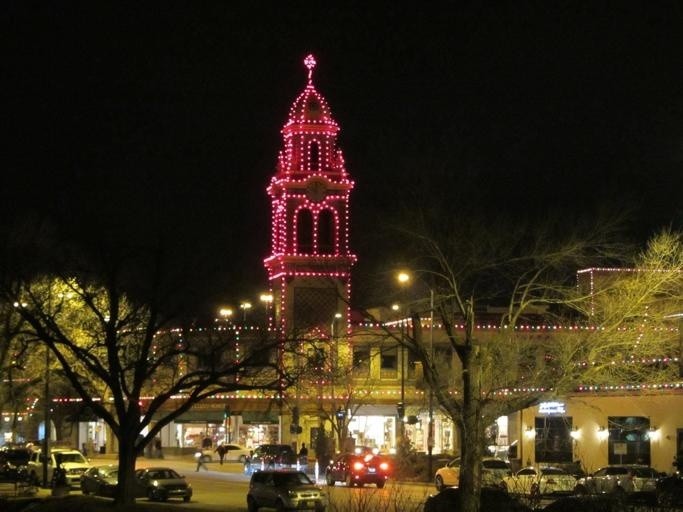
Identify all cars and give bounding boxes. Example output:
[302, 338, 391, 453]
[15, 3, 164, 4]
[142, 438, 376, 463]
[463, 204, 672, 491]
[188, 443, 254, 463]
[241, 467, 332, 511]
[501, 462, 577, 497]
[324, 446, 389, 488]
[77, 457, 192, 503]
[573, 464, 664, 496]
[422, 486, 626, 512]
[431, 457, 516, 491]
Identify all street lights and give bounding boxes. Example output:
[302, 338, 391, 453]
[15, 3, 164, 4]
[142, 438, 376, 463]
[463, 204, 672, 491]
[396, 269, 435, 477]
[328, 310, 343, 447]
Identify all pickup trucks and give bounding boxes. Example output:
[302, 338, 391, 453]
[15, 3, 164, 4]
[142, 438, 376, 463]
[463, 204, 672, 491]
[247, 443, 309, 474]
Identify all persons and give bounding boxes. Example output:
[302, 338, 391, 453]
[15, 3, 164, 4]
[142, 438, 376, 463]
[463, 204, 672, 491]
[296, 443, 308, 473]
[192, 447, 208, 472]
[214, 441, 226, 465]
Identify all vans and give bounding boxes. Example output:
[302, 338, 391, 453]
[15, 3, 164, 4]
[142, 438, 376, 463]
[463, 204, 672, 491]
[28, 446, 88, 490]
